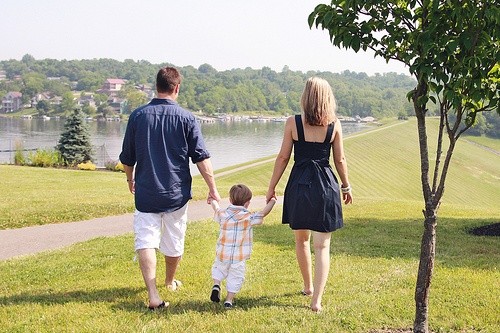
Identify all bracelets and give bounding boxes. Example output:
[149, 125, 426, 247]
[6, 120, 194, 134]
[341, 184, 352, 193]
[126, 178, 136, 184]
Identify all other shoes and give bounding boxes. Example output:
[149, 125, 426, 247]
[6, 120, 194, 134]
[224, 303, 233, 310]
[210, 285, 220, 302]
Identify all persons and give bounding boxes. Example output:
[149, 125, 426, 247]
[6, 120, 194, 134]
[119, 66, 220, 310]
[206, 184, 277, 310]
[265, 77, 353, 313]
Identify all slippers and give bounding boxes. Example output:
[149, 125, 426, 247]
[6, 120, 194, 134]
[302, 289, 313, 296]
[172, 280, 181, 290]
[149, 301, 169, 310]
